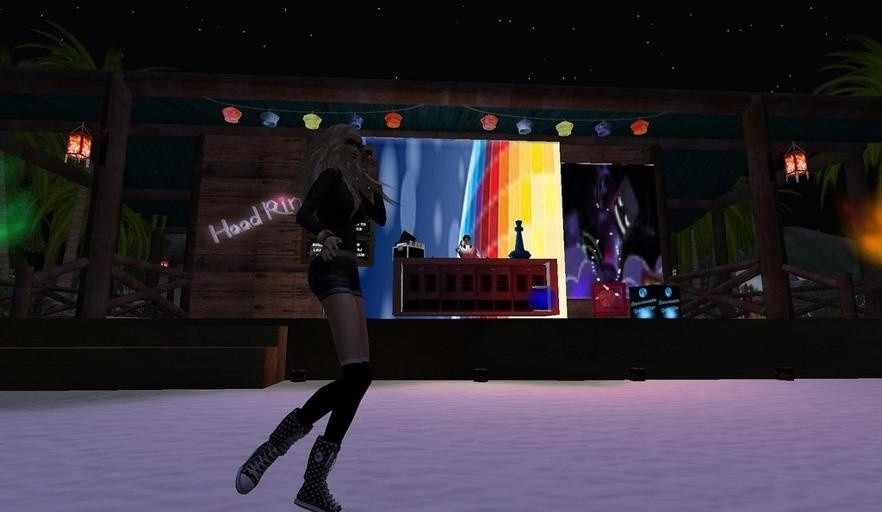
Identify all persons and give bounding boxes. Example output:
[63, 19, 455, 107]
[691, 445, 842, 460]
[621, 248, 661, 285]
[455, 235, 482, 258]
[236, 121, 386, 511]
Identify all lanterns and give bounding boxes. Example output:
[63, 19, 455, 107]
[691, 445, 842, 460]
[783, 143, 810, 183]
[64, 122, 93, 169]
